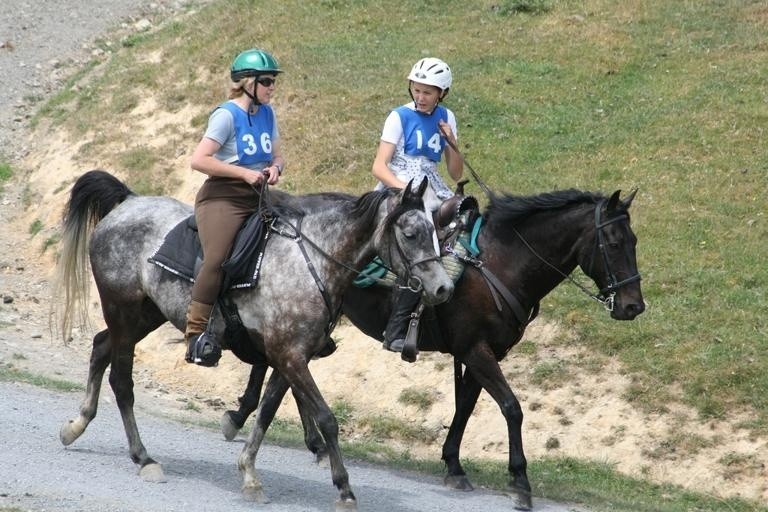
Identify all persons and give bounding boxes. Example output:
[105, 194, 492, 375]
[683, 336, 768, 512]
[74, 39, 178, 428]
[368, 57, 467, 353]
[181, 46, 337, 365]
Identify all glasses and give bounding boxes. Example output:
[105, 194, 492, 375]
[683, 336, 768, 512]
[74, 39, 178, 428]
[255, 77, 275, 87]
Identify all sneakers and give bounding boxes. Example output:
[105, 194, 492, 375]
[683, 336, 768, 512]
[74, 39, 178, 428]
[383, 330, 419, 354]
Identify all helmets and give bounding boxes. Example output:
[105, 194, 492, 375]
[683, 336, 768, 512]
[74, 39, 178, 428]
[231, 49, 284, 82]
[407, 57, 453, 102]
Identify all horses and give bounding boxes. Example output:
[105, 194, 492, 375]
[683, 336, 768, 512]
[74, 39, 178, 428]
[218, 186, 646, 512]
[47, 168, 456, 512]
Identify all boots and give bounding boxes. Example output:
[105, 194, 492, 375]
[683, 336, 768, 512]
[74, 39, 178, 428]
[185, 299, 214, 360]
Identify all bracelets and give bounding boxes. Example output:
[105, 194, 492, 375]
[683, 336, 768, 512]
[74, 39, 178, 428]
[273, 163, 282, 177]
